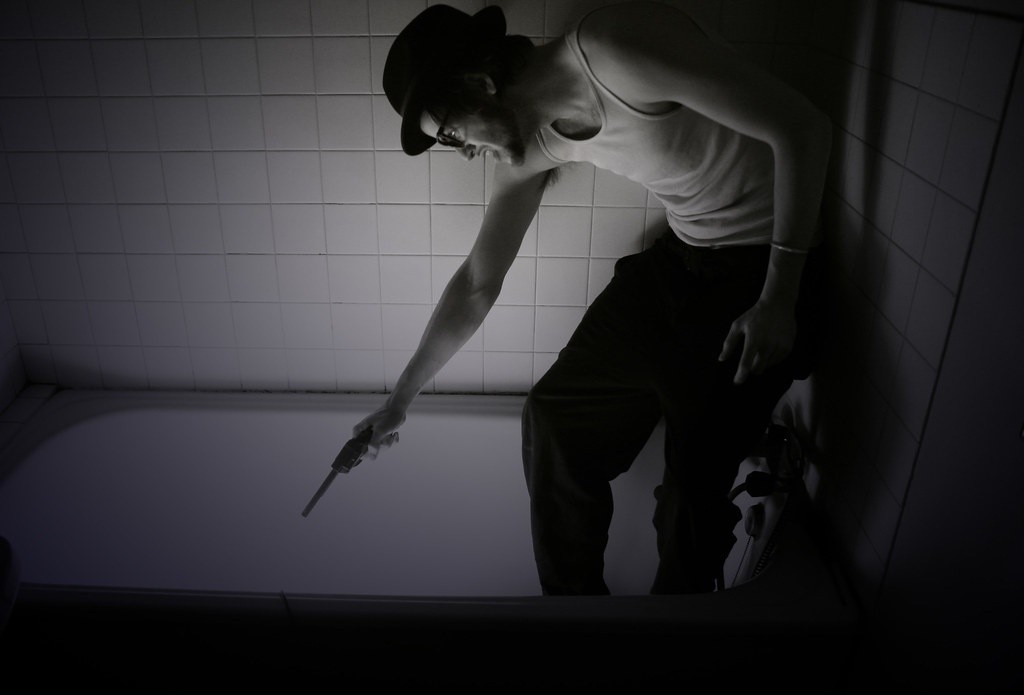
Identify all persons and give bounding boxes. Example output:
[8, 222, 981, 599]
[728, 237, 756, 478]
[353, 1, 837, 596]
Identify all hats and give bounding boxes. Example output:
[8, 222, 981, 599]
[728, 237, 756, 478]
[382, 5, 507, 157]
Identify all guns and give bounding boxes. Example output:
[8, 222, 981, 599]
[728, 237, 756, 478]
[301, 425, 400, 519]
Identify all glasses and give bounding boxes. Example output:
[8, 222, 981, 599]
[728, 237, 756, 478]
[436, 77, 464, 148]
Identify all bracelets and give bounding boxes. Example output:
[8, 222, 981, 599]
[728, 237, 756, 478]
[770, 240, 811, 259]
